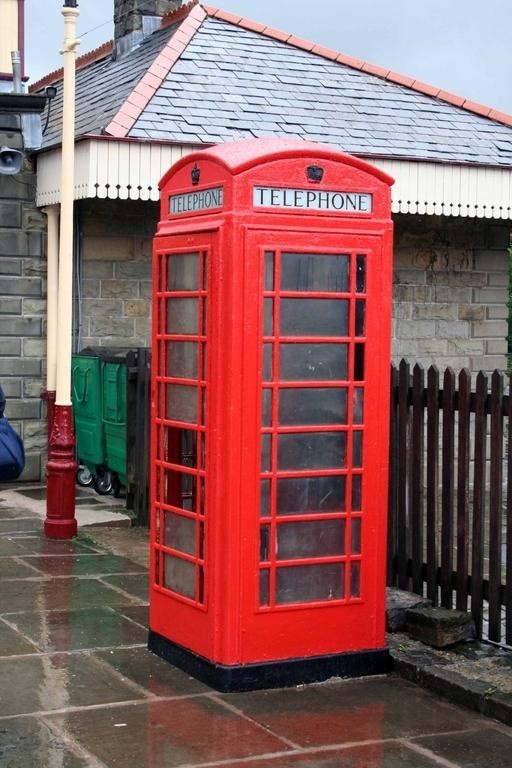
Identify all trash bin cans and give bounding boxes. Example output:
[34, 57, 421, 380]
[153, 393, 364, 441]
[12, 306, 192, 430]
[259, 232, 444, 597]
[72, 354, 120, 497]
[93, 352, 137, 497]
